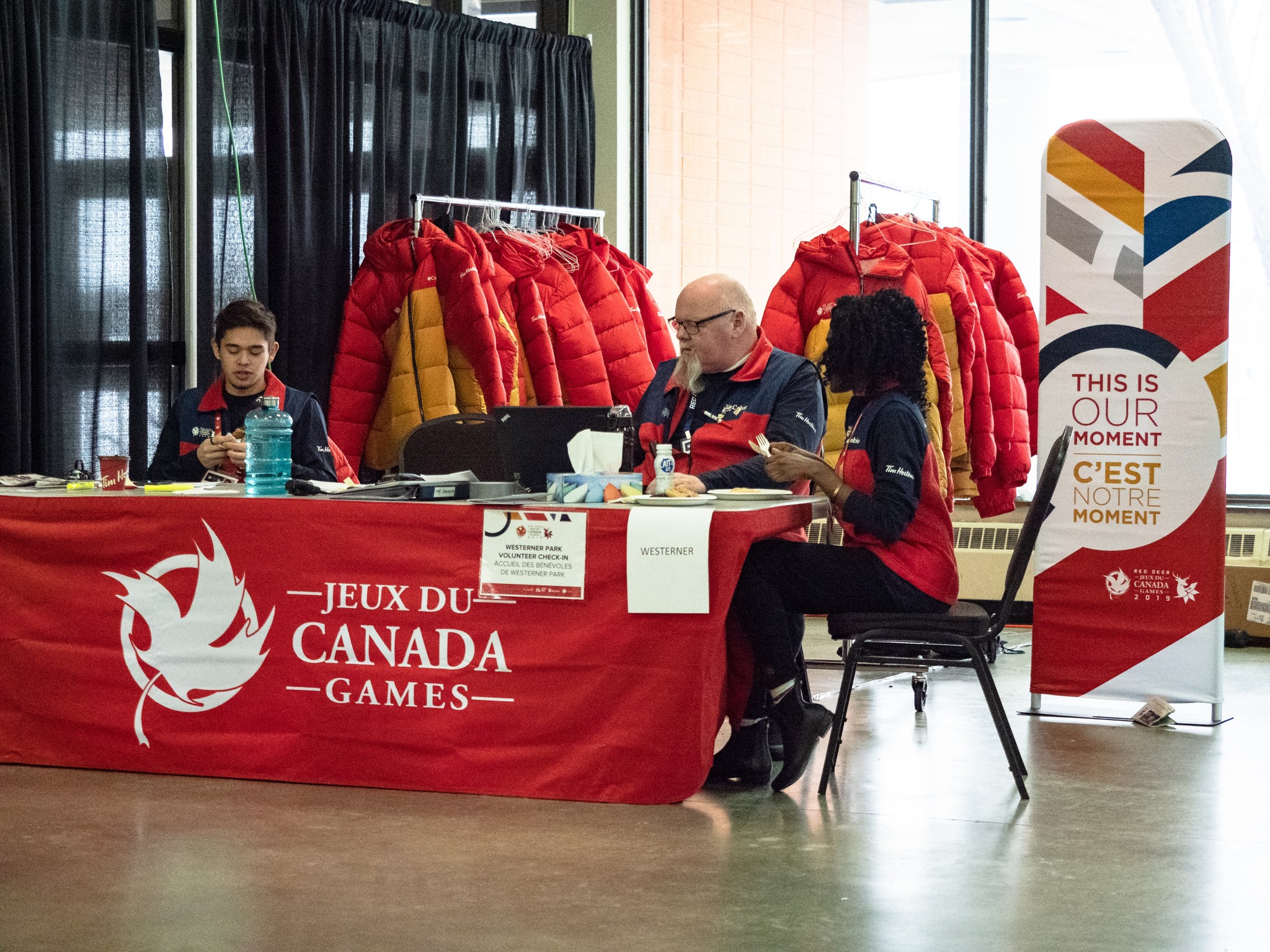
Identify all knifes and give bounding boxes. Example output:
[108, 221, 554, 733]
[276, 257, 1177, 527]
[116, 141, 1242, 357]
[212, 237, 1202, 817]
[749, 440, 768, 456]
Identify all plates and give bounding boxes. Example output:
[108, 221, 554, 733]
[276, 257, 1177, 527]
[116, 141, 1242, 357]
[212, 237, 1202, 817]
[636, 495, 716, 505]
[707, 489, 793, 500]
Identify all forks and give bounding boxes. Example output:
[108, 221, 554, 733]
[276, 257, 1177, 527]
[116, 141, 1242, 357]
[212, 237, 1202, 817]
[756, 434, 771, 457]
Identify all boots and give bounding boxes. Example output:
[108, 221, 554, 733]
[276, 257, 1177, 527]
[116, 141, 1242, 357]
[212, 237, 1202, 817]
[707, 716, 772, 784]
[768, 677, 835, 790]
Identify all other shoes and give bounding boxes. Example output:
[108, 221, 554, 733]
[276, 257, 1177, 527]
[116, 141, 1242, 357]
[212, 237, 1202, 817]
[766, 709, 783, 753]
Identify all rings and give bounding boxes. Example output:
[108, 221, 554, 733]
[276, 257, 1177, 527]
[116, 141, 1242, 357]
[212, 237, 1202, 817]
[210, 437, 213, 444]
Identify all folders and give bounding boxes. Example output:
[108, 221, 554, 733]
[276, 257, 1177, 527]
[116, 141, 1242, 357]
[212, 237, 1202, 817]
[330, 479, 469, 500]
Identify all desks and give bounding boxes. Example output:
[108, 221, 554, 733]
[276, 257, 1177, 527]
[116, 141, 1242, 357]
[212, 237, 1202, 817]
[0, 478, 831, 804]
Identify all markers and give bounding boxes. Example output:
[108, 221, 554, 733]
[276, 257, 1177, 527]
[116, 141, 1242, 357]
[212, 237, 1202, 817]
[67, 481, 96, 490]
[145, 485, 192, 491]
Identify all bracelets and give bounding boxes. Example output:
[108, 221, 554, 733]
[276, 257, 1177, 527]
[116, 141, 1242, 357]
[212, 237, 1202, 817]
[830, 483, 843, 504]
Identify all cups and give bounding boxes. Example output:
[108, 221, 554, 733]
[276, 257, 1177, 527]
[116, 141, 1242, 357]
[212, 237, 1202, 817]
[99, 456, 131, 491]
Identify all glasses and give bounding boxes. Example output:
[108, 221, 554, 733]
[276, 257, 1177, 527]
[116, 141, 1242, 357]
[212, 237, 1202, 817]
[667, 308, 736, 335]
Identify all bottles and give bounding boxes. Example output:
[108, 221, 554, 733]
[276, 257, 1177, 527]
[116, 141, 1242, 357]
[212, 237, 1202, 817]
[654, 445, 675, 493]
[245, 397, 294, 495]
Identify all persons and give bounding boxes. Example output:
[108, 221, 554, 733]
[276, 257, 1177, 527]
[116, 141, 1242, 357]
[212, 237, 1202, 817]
[146, 300, 338, 483]
[706, 287, 959, 793]
[632, 275, 825, 542]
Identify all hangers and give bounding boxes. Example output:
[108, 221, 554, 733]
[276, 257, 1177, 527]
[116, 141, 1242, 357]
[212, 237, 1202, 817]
[348, 193, 646, 295]
[793, 170, 1008, 284]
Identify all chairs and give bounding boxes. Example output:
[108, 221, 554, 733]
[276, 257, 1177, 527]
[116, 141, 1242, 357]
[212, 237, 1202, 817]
[399, 414, 495, 481]
[819, 425, 1074, 800]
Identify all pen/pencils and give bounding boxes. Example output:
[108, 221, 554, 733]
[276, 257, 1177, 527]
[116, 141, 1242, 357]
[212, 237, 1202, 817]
[126, 486, 144, 490]
[703, 410, 733, 429]
